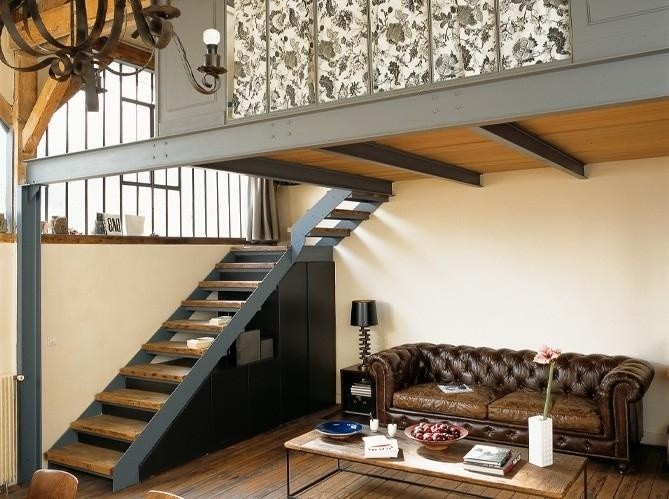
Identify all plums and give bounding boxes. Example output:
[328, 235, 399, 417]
[414, 422, 459, 440]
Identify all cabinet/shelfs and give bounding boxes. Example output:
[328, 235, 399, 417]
[340, 363, 378, 419]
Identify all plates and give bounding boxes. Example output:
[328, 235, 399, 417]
[314, 420, 364, 442]
[404, 421, 469, 451]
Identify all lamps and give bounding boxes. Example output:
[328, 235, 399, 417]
[0, 0, 226, 94]
[350, 300, 378, 370]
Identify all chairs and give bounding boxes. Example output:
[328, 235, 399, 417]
[24, 469, 79, 499]
[145, 490, 184, 499]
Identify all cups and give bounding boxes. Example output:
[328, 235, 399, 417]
[369, 418, 379, 434]
[387, 424, 397, 438]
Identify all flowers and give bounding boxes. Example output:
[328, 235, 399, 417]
[534, 345, 561, 420]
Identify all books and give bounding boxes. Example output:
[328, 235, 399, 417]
[463, 444, 512, 465]
[462, 449, 521, 476]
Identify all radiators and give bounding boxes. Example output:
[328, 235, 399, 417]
[0, 374, 24, 495]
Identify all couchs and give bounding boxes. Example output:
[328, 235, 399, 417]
[363, 343, 655, 474]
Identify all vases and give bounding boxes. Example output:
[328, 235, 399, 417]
[528, 416, 554, 468]
